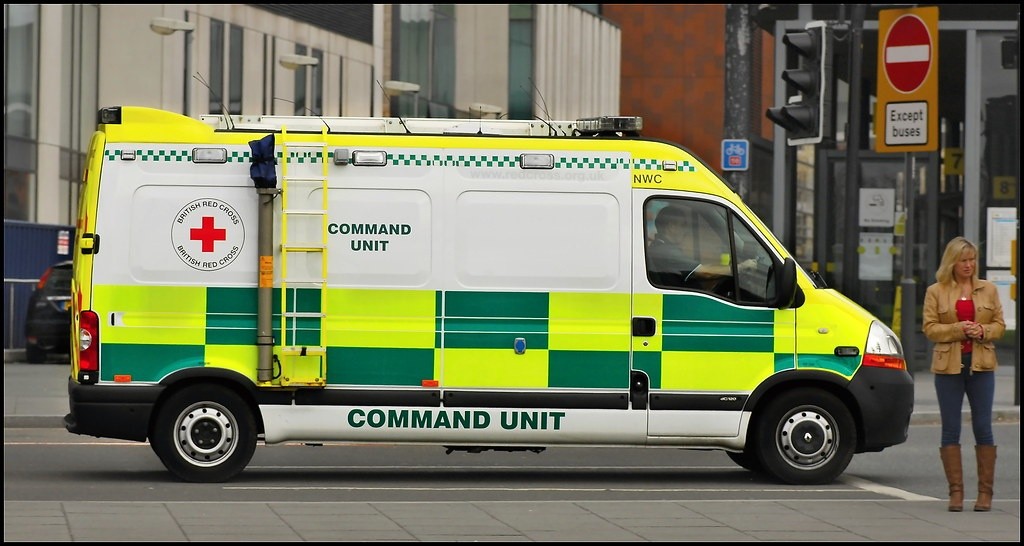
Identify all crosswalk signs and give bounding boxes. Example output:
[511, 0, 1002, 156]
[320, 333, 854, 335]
[720, 139, 750, 171]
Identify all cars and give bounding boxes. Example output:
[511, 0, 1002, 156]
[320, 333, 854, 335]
[26, 259, 74, 363]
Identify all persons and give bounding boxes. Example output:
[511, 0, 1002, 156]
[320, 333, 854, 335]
[645, 206, 757, 288]
[922, 237, 1006, 512]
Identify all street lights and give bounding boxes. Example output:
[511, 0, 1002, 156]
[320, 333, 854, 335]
[279, 53, 319, 115]
[150, 16, 196, 116]
[384, 79, 421, 118]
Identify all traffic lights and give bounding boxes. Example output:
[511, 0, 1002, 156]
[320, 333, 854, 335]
[765, 20, 834, 146]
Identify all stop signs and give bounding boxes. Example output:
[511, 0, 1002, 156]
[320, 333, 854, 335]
[883, 14, 934, 94]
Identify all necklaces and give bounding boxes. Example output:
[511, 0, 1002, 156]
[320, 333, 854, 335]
[960, 286, 973, 301]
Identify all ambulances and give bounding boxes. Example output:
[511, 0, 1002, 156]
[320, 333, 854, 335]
[64, 104, 915, 484]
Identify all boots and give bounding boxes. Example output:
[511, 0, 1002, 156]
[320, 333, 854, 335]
[974, 445, 997, 511]
[939, 444, 964, 512]
